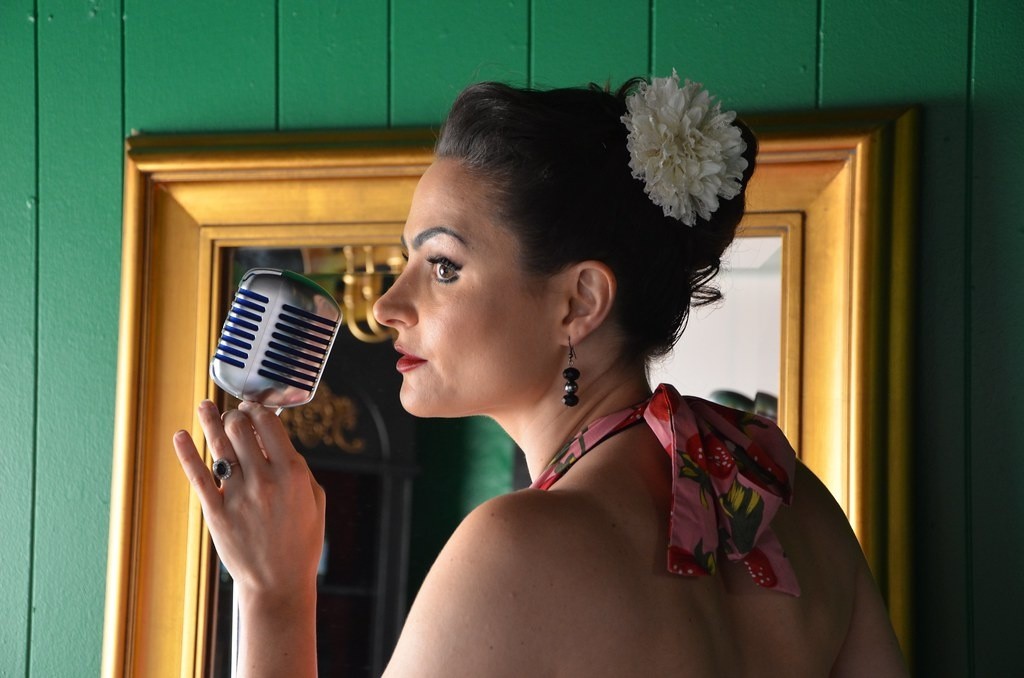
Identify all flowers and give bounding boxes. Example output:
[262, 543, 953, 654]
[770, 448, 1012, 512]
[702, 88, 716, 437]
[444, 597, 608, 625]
[619, 66, 750, 228]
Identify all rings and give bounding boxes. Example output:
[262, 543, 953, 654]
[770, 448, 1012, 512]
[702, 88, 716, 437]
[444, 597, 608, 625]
[212, 459, 239, 481]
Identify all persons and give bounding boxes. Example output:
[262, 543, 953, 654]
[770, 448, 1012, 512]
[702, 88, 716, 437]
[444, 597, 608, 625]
[172, 80, 905, 678]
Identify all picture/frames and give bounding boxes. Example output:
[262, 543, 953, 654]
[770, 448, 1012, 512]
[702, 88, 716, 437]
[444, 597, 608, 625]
[97, 96, 917, 678]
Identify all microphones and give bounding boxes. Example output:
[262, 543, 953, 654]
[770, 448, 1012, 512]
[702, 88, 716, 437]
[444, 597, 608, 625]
[210, 266, 343, 429]
